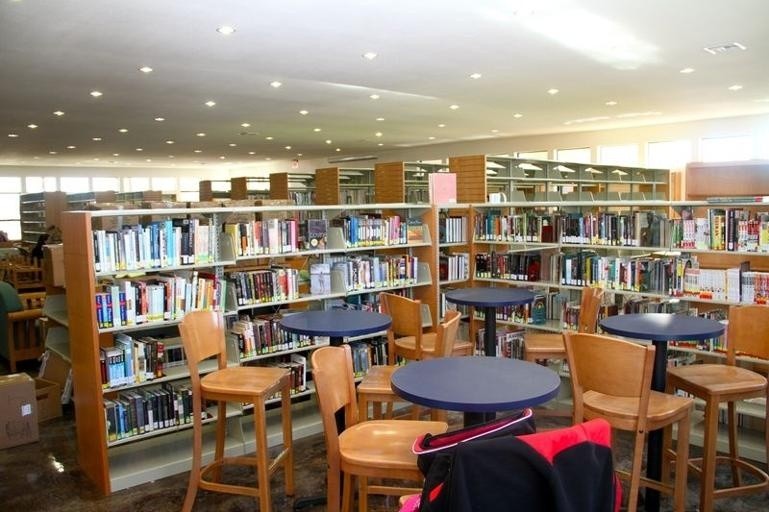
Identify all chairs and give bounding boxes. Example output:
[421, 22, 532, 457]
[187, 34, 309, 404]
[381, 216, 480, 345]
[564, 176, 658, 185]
[561, 329, 695, 512]
[402, 419, 615, 511]
[382, 292, 475, 360]
[523, 289, 607, 364]
[667, 307, 769, 512]
[179, 312, 295, 511]
[310, 346, 450, 512]
[358, 311, 461, 420]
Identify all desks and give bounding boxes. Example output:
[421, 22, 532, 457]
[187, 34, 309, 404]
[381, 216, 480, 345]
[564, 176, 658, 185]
[445, 287, 535, 356]
[393, 353, 563, 420]
[600, 313, 728, 512]
[278, 310, 394, 511]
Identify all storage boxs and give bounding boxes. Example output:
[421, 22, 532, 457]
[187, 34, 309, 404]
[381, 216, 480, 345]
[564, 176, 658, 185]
[36, 378, 63, 424]
[0, 373, 41, 450]
[90, 203, 188, 232]
[42, 244, 64, 292]
[190, 201, 297, 225]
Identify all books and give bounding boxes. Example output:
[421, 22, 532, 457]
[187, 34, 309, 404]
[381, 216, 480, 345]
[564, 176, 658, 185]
[667, 350, 704, 399]
[475, 325, 524, 361]
[429, 173, 457, 205]
[440, 287, 468, 317]
[223, 218, 328, 257]
[536, 358, 571, 373]
[440, 216, 467, 243]
[407, 188, 429, 203]
[92, 218, 222, 273]
[475, 207, 769, 252]
[226, 264, 332, 304]
[100, 333, 165, 389]
[96, 272, 227, 328]
[475, 281, 582, 330]
[231, 313, 329, 359]
[346, 289, 411, 313]
[240, 354, 306, 407]
[332, 254, 417, 292]
[331, 212, 425, 247]
[706, 196, 768, 203]
[454, 321, 470, 342]
[102, 383, 206, 440]
[439, 252, 470, 280]
[596, 290, 740, 356]
[290, 192, 317, 206]
[476, 250, 769, 305]
[350, 338, 406, 378]
